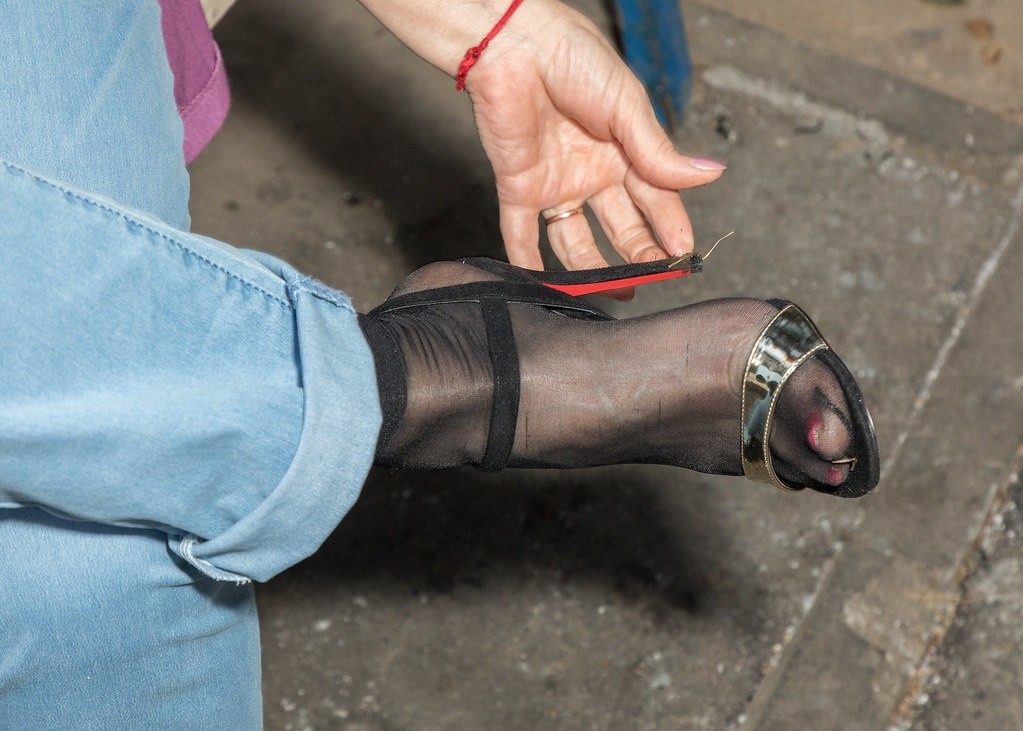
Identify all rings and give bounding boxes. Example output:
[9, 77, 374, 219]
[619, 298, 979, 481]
[544, 208, 587, 227]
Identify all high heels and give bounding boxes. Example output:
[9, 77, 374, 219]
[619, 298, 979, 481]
[368, 252, 881, 497]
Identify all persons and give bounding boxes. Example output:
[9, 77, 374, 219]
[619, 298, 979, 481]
[0, 0, 879, 731]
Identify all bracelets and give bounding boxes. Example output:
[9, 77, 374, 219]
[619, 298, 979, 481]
[454, 0, 523, 91]
